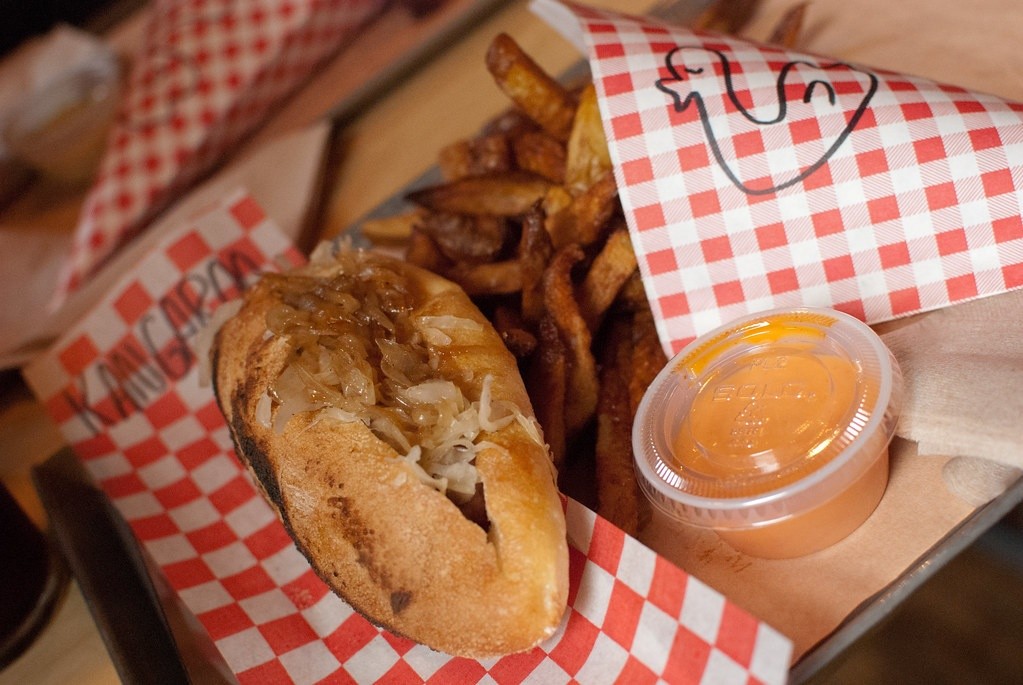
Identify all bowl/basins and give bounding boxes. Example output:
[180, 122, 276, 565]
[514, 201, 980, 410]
[632, 307, 904, 559]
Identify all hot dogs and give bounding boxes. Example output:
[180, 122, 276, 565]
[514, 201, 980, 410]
[210, 235, 569, 657]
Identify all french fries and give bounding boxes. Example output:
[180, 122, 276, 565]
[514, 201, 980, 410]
[354, 31, 672, 534]
